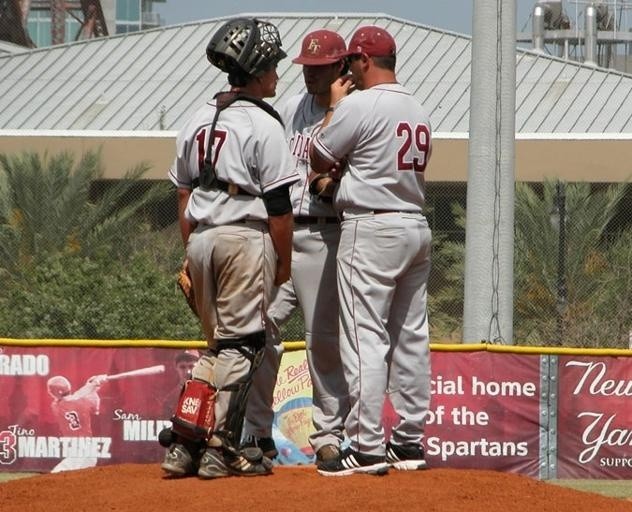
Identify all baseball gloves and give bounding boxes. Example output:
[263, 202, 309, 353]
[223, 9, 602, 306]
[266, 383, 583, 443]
[178, 261, 197, 319]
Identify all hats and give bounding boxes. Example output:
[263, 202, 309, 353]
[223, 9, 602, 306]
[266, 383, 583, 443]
[172, 346, 202, 367]
[290, 29, 348, 67]
[327, 25, 397, 59]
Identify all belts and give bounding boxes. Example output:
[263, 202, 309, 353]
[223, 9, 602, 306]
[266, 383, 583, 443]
[294, 212, 342, 230]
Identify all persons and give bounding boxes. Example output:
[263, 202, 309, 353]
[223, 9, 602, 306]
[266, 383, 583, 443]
[242, 30, 366, 468]
[310, 27, 433, 477]
[160, 19, 292, 478]
[163, 348, 200, 418]
[48, 375, 105, 474]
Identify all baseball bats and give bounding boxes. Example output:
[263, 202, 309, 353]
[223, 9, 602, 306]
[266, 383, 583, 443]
[89, 364, 167, 385]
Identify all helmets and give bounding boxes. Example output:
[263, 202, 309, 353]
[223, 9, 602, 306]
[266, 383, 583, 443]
[205, 16, 289, 85]
[46, 374, 73, 402]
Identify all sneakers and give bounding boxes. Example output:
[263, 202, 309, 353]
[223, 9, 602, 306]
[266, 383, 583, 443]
[314, 446, 393, 479]
[196, 429, 279, 480]
[158, 430, 195, 477]
[384, 439, 432, 474]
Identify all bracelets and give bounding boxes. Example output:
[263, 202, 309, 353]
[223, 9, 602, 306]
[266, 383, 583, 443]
[324, 107, 333, 115]
